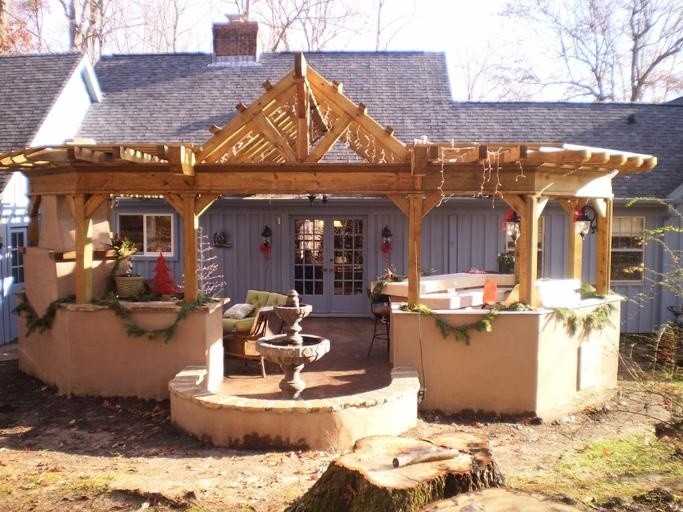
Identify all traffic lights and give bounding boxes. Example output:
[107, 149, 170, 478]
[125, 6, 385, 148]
[223, 304, 255, 319]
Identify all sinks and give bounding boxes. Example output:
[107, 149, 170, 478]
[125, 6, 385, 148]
[223, 289, 302, 334]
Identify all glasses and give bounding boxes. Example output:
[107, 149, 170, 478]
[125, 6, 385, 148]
[575, 204, 598, 240]
[382, 225, 392, 245]
[261, 225, 272, 246]
[505, 210, 521, 243]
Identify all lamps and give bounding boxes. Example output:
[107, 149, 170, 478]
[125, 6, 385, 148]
[223, 307, 274, 378]
[365, 287, 391, 361]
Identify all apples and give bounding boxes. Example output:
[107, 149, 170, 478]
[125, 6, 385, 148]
[109, 231, 113, 239]
